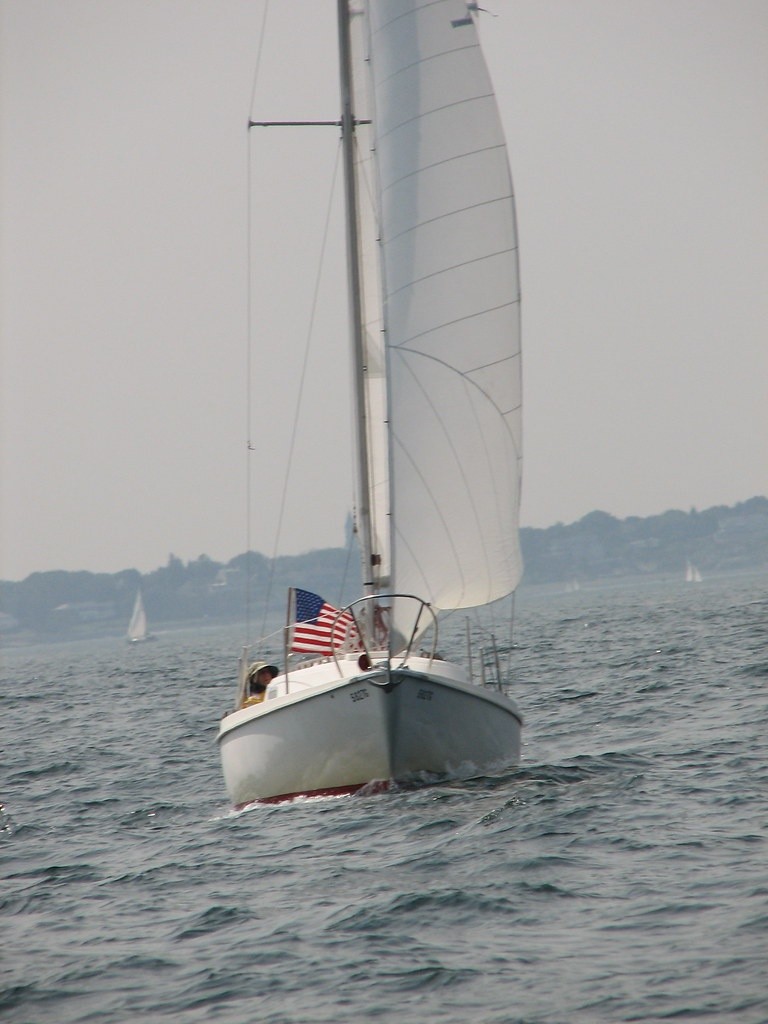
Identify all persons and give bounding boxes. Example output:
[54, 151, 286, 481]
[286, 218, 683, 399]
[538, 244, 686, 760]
[243, 662, 278, 708]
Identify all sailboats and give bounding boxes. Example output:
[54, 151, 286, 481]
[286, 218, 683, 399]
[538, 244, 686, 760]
[125, 588, 155, 644]
[216, 0, 528, 808]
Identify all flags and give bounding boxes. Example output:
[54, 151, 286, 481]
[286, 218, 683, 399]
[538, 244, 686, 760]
[291, 590, 356, 655]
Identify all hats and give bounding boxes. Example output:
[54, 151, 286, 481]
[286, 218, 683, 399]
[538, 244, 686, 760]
[250, 664, 279, 679]
